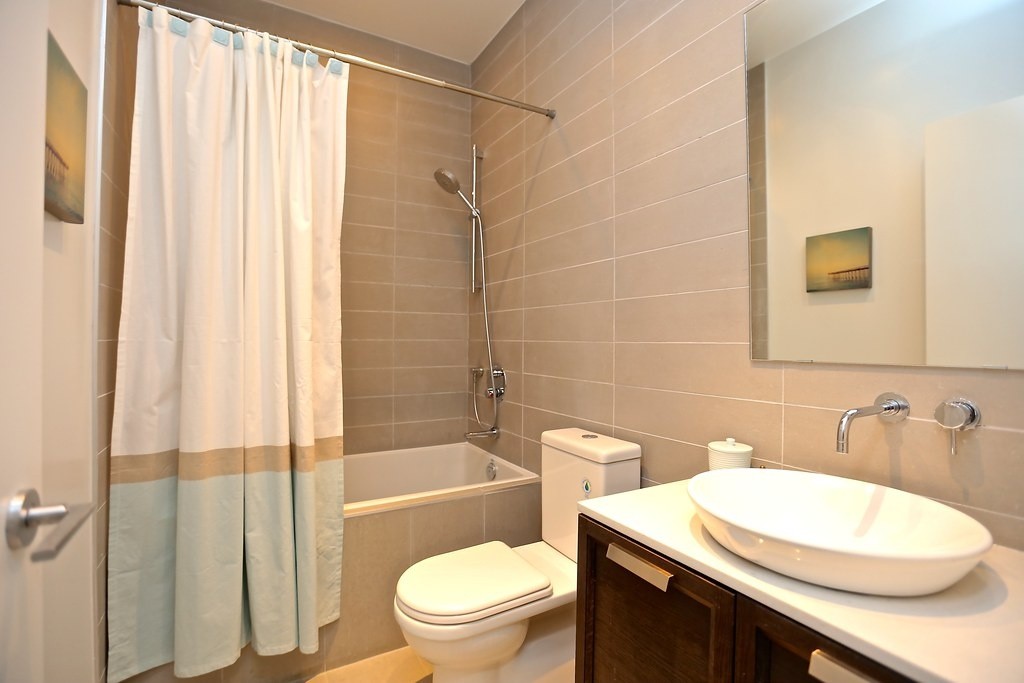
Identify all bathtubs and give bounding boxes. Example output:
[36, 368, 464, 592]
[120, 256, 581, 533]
[107, 439, 541, 682]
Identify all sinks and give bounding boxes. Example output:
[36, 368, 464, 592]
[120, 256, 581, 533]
[687, 467, 994, 597]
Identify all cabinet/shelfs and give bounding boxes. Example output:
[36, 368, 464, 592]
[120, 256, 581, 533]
[575, 512, 919, 683]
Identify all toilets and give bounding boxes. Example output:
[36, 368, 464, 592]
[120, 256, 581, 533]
[392, 426, 642, 683]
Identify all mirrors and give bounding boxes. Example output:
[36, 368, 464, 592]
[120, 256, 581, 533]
[743, 0, 1024, 370]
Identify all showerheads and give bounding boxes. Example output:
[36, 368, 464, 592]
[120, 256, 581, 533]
[433, 165, 483, 220]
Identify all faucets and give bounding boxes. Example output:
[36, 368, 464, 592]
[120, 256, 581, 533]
[836, 392, 911, 455]
